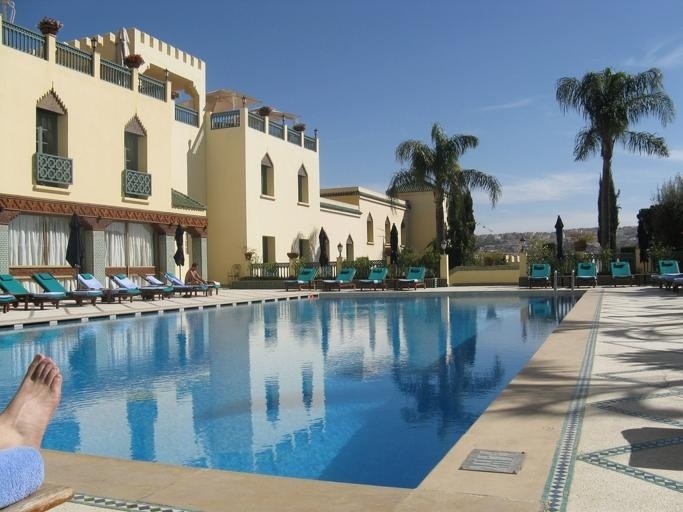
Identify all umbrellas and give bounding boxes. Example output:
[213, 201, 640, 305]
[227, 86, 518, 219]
[184, 88, 262, 109]
[248, 103, 278, 117]
[317, 302, 328, 361]
[553, 215, 564, 267]
[177, 314, 186, 365]
[636, 220, 650, 272]
[391, 299, 400, 359]
[172, 222, 184, 280]
[65, 210, 83, 289]
[317, 227, 329, 280]
[270, 109, 301, 122]
[389, 223, 400, 279]
[114, 27, 133, 68]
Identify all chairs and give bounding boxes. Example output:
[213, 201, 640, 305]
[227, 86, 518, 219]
[282, 266, 430, 291]
[1, 271, 225, 312]
[521, 260, 683, 289]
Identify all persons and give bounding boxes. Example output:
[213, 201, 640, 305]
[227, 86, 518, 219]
[0, 352, 64, 453]
[184, 263, 209, 285]
